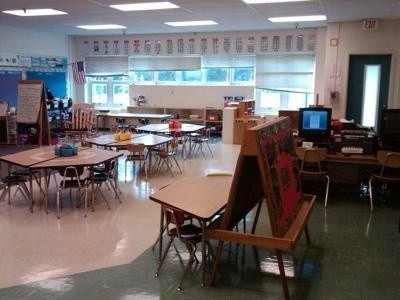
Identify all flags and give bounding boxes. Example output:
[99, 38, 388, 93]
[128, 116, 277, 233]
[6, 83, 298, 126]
[72, 61, 88, 87]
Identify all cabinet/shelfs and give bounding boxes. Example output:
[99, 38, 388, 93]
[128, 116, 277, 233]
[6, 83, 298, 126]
[221, 98, 267, 144]
[127, 105, 223, 137]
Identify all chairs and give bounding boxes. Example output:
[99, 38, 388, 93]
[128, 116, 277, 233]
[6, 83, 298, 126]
[295, 146, 400, 213]
[61, 103, 96, 146]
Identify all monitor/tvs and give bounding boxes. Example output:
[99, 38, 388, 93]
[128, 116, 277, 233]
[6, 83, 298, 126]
[299, 108, 332, 136]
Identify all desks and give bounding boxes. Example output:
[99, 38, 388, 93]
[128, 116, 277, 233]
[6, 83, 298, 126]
[94, 113, 172, 135]
